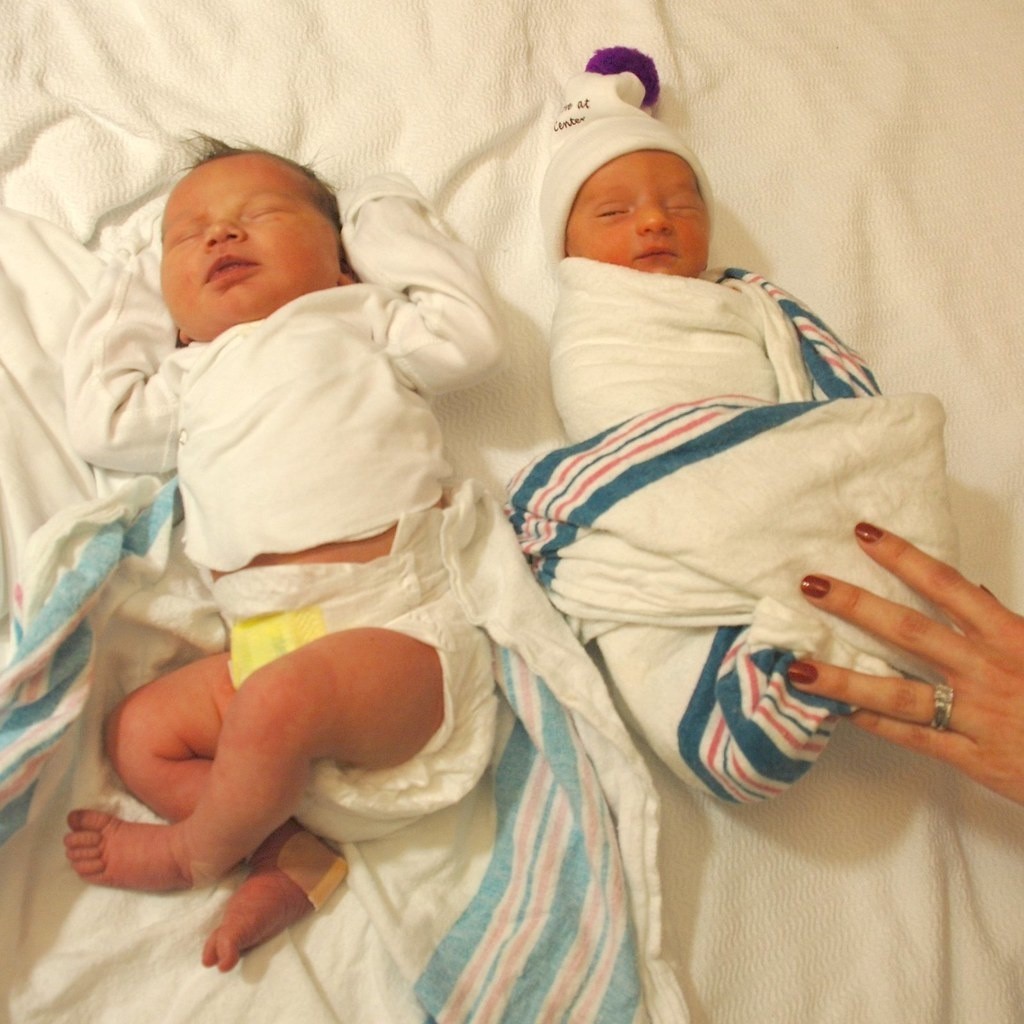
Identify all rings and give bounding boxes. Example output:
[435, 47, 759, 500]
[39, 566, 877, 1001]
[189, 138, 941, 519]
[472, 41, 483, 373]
[929, 683, 954, 732]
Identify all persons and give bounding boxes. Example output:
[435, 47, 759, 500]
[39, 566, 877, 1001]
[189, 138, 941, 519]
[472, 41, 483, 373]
[64, 126, 508, 972]
[502, 45, 957, 802]
[787, 521, 1024, 809]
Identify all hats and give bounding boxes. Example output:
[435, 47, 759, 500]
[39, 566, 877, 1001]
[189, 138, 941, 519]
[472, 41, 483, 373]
[538, 46, 715, 285]
